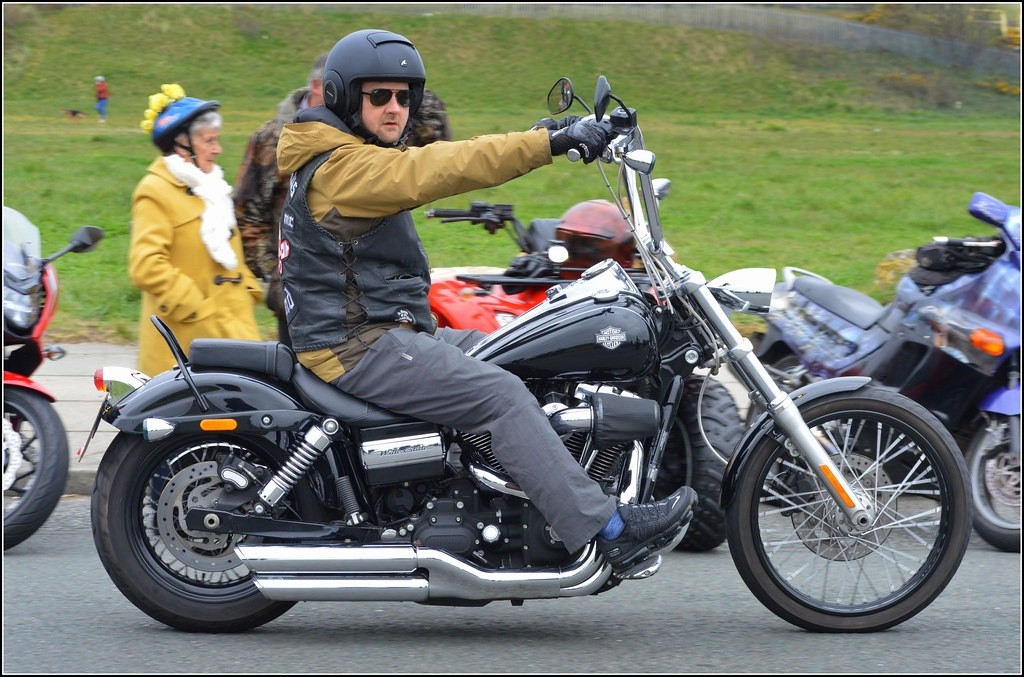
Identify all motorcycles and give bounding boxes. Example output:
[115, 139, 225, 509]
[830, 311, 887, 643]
[86, 74, 975, 635]
[745, 191, 1021, 553]
[3, 205, 106, 553]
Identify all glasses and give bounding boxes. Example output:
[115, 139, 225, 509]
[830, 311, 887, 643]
[361, 88, 416, 108]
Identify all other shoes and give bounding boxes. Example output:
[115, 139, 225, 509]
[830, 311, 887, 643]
[99, 118, 105, 123]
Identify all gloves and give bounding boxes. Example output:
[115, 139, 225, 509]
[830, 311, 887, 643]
[548, 114, 607, 164]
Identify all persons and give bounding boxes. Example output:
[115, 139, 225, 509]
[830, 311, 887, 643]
[127, 82, 264, 535]
[94, 74, 110, 124]
[280, 28, 697, 574]
[232, 49, 453, 342]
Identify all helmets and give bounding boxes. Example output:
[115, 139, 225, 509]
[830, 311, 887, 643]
[552, 198, 635, 279]
[323, 29, 425, 122]
[139, 82, 222, 154]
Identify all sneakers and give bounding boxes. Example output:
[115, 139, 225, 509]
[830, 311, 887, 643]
[597, 484, 699, 574]
